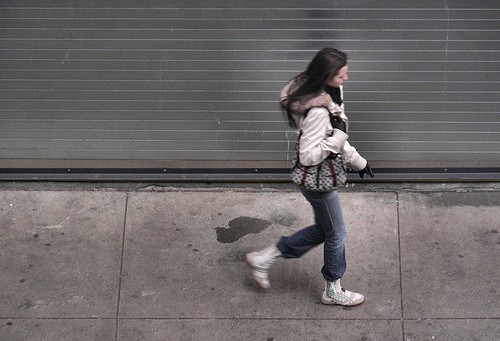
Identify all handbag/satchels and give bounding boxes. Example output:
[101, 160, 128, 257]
[290, 105, 347, 193]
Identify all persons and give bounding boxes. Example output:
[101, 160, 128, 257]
[246, 48, 373, 306]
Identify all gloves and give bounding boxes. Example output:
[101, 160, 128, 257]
[359, 165, 373, 178]
[330, 115, 346, 133]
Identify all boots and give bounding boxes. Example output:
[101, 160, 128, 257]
[321, 278, 364, 306]
[246, 245, 282, 290]
